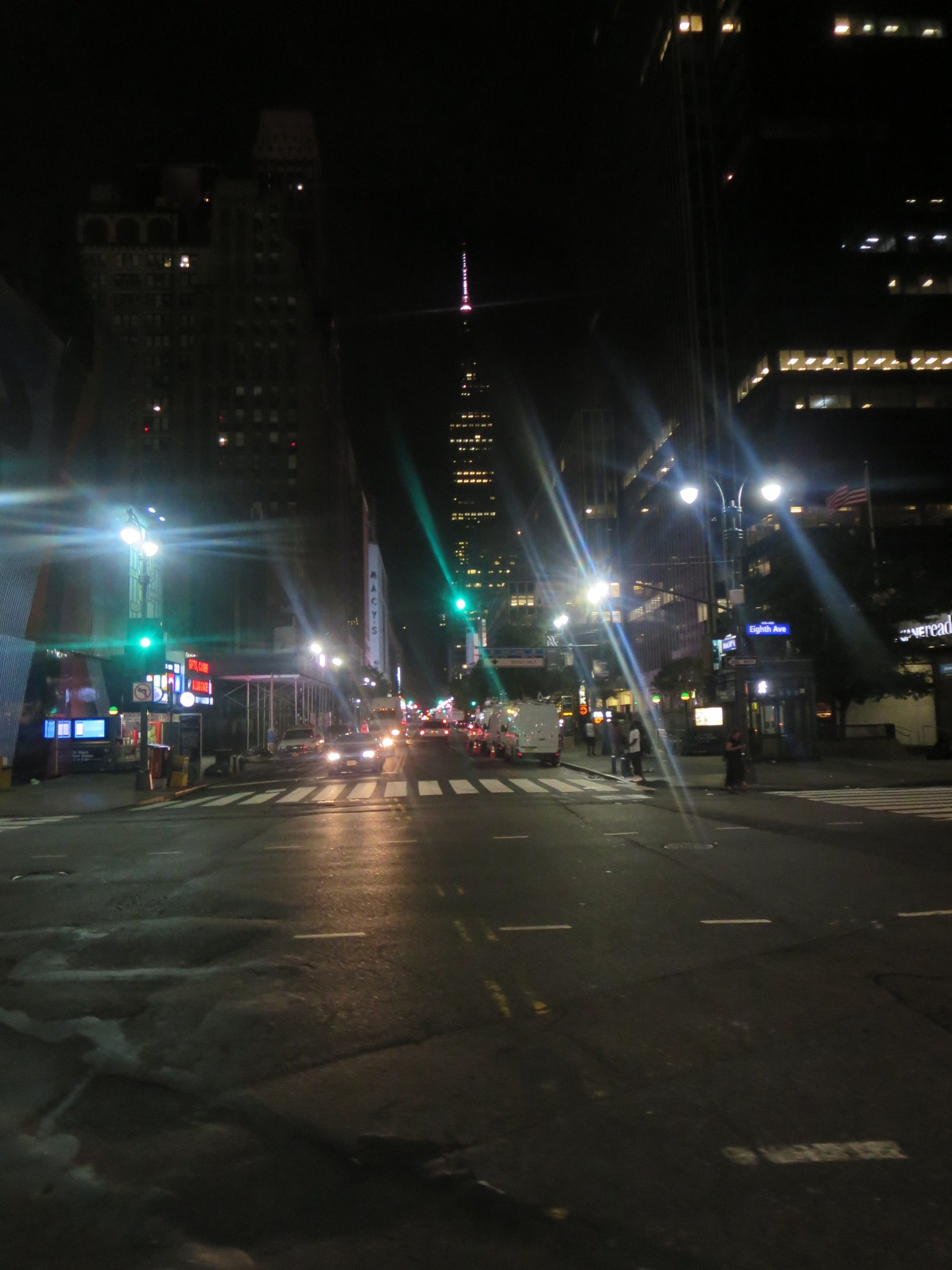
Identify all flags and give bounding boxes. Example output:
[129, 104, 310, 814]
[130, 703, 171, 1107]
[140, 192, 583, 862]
[825, 475, 868, 514]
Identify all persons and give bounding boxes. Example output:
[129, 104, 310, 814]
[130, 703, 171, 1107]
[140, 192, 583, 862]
[628, 721, 643, 781]
[725, 729, 748, 787]
[586, 720, 595, 756]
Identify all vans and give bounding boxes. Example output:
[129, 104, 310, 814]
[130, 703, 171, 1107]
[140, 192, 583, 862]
[474, 700, 562, 766]
[435, 710, 464, 727]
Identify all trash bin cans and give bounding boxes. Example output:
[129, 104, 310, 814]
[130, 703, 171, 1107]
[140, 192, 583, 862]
[169, 755, 189, 788]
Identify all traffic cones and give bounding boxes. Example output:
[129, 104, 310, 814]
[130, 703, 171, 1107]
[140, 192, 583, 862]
[472, 742, 477, 754]
[490, 746, 496, 760]
[476, 743, 481, 755]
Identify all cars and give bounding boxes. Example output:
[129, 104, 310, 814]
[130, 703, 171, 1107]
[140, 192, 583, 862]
[369, 726, 396, 757]
[324, 733, 385, 776]
[277, 724, 324, 759]
[419, 719, 450, 739]
[323, 725, 357, 739]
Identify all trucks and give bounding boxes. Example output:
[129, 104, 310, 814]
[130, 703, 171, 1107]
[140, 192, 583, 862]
[361, 696, 407, 738]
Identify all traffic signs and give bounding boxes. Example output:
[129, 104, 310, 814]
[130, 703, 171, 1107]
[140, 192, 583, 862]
[722, 656, 759, 669]
[576, 681, 589, 718]
[484, 658, 544, 668]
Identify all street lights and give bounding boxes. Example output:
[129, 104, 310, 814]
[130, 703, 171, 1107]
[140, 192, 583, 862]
[652, 695, 661, 749]
[310, 642, 322, 728]
[680, 693, 690, 755]
[108, 705, 118, 773]
[675, 463, 784, 787]
[119, 509, 160, 790]
[588, 581, 615, 690]
